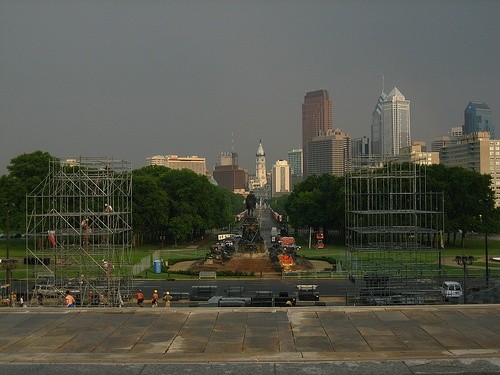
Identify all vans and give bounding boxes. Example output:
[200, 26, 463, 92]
[225, 241, 233, 246]
[441, 281, 464, 299]
[233, 233, 242, 237]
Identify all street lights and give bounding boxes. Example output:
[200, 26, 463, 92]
[160, 236, 165, 250]
[1, 233, 26, 307]
[456, 254, 474, 305]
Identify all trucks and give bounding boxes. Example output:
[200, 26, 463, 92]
[282, 243, 301, 251]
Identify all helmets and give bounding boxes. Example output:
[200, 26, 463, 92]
[102, 260, 104, 262]
[105, 204, 107, 206]
[86, 219, 89, 222]
[154, 290, 157, 293]
[166, 292, 169, 295]
[139, 290, 141, 292]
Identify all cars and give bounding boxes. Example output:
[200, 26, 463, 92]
[316, 241, 325, 249]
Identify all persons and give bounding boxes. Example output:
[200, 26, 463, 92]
[65, 290, 75, 307]
[20, 296, 24, 307]
[164, 292, 173, 308]
[151, 289, 160, 306]
[81, 219, 88, 229]
[136, 289, 144, 304]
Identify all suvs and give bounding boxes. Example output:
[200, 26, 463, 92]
[31, 285, 66, 299]
